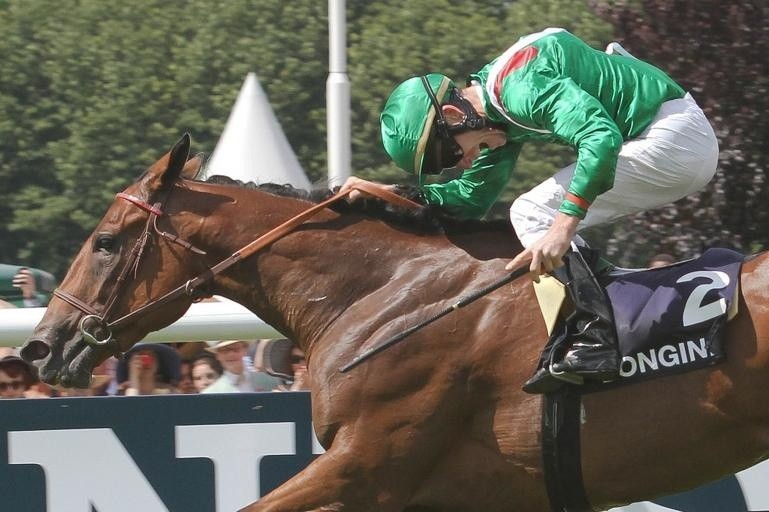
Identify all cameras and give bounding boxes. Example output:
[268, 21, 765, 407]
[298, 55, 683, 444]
[141, 355, 153, 365]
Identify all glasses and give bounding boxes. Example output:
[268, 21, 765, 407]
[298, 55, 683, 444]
[289, 355, 306, 364]
[421, 75, 464, 168]
[0, 381, 23, 390]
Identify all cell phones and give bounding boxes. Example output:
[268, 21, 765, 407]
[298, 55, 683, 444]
[18, 267, 27, 284]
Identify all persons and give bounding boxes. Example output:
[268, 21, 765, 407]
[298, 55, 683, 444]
[647, 253, 679, 271]
[340, 25, 720, 396]
[0, 266, 307, 399]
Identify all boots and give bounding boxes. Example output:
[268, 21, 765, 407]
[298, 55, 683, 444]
[522, 251, 620, 394]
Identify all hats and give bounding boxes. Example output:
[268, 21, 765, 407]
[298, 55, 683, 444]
[205, 339, 251, 354]
[117, 343, 182, 390]
[257, 340, 301, 382]
[0, 347, 39, 387]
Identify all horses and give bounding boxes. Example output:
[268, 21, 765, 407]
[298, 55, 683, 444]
[14, 127, 769, 512]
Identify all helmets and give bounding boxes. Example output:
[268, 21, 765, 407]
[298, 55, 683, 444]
[380, 73, 454, 176]
